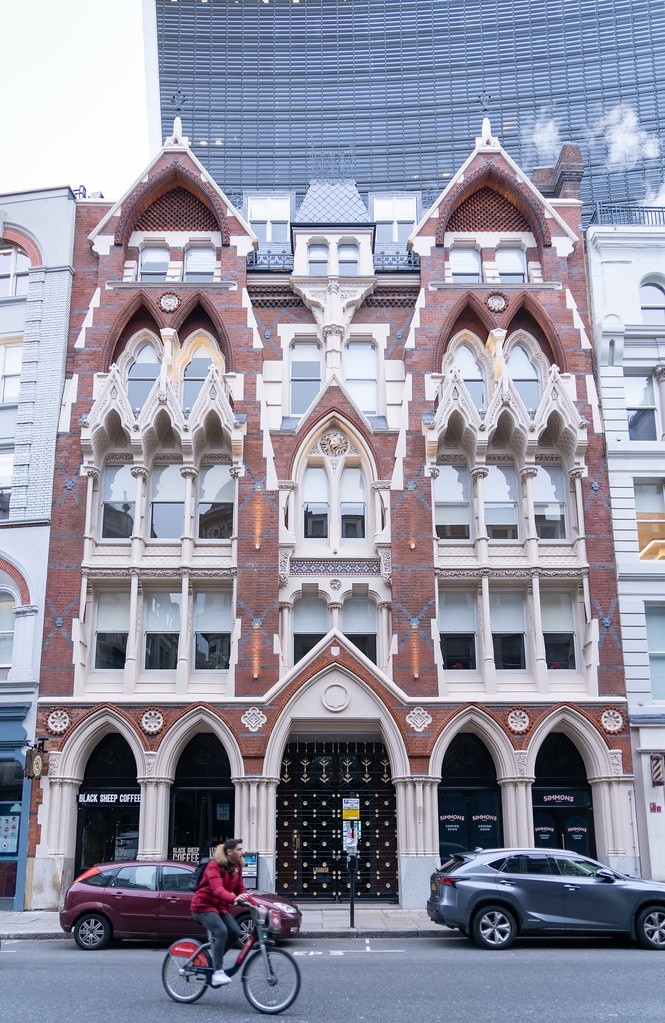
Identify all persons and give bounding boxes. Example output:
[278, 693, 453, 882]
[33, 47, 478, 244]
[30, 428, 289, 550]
[191, 839, 259, 986]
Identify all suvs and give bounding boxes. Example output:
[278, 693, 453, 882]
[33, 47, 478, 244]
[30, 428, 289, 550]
[427, 848, 665, 950]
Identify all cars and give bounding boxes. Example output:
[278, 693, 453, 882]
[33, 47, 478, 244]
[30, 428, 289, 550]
[59, 862, 302, 950]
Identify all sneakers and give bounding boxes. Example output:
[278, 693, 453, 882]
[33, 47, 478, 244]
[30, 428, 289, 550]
[212, 972, 232, 986]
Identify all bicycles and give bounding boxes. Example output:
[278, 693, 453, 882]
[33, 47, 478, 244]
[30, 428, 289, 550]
[161, 889, 301, 1015]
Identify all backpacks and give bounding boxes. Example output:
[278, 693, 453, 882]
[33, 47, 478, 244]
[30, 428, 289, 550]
[187, 858, 225, 893]
